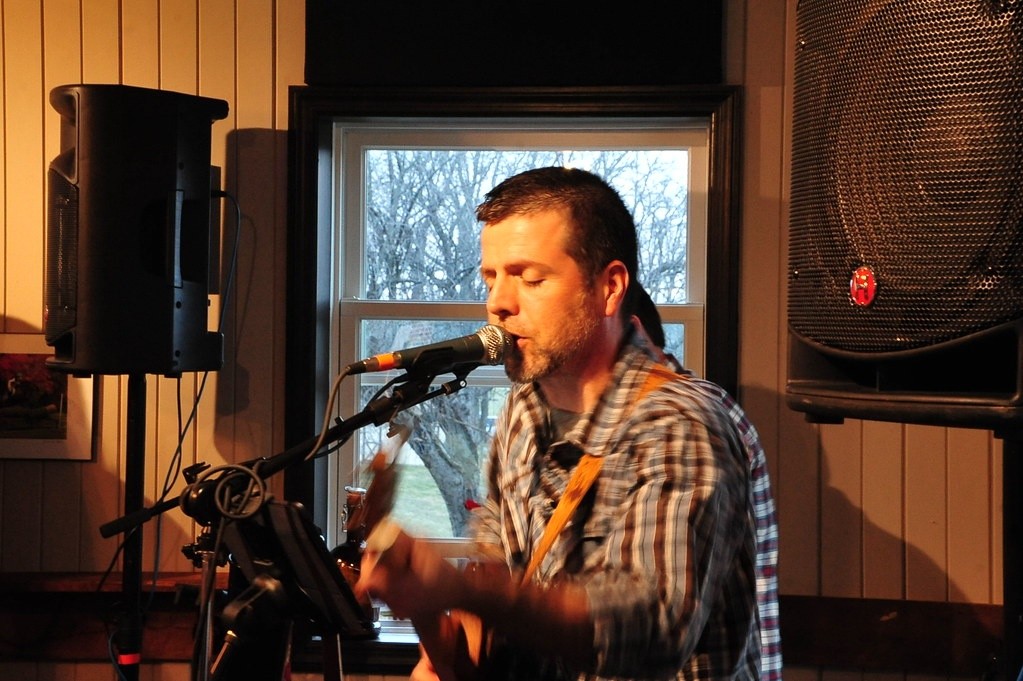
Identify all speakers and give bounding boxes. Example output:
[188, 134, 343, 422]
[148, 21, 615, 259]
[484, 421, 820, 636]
[44, 84, 229, 378]
[785, 0, 1023, 439]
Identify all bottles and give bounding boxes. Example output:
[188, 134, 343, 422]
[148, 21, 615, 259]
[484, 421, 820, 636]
[330, 486, 382, 639]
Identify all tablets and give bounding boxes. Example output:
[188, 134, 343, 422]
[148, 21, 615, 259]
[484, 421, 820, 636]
[221, 499, 377, 643]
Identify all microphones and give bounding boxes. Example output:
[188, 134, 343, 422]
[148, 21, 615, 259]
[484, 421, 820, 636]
[346, 325, 514, 376]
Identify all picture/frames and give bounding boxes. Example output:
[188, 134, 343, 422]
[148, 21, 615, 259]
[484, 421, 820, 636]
[0, 331, 98, 463]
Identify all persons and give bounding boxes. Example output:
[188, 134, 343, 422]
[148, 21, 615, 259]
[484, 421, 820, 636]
[354, 167, 784, 681]
